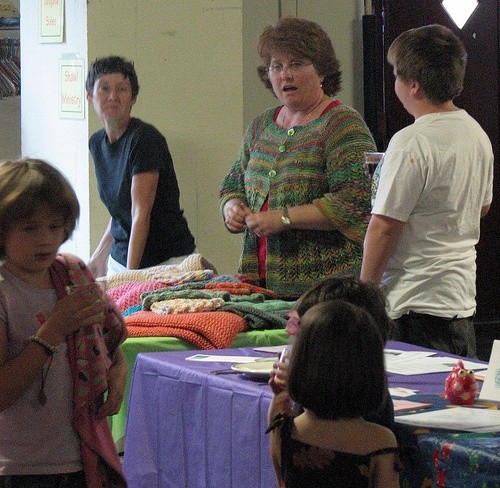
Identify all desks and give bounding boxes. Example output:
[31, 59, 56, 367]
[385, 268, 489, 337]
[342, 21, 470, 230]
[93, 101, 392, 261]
[123, 340, 500, 488]
[103, 328, 297, 454]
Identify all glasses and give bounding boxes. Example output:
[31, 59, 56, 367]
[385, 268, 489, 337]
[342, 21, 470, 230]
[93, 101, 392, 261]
[270, 60, 313, 74]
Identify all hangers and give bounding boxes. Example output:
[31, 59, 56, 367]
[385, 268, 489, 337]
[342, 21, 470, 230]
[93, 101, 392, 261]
[0, 36, 21, 99]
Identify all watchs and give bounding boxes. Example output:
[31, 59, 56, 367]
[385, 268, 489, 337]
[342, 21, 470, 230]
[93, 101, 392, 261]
[281, 209, 291, 230]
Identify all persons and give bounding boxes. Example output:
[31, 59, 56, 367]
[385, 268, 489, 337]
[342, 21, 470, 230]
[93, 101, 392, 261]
[265, 276, 401, 488]
[85, 56, 197, 277]
[0, 157, 128, 488]
[360, 25, 494, 358]
[219, 17, 377, 302]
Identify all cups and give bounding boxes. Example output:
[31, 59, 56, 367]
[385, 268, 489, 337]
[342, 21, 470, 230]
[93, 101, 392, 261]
[65, 280, 106, 322]
[365, 152, 385, 178]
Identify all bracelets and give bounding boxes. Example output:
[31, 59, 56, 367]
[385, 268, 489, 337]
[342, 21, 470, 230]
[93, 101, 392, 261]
[29, 335, 59, 353]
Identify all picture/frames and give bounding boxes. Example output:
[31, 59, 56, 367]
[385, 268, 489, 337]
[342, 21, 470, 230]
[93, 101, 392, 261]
[37, 0, 64, 43]
[57, 59, 84, 119]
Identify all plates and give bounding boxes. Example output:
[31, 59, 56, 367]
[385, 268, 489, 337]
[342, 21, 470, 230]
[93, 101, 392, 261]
[231, 356, 278, 379]
